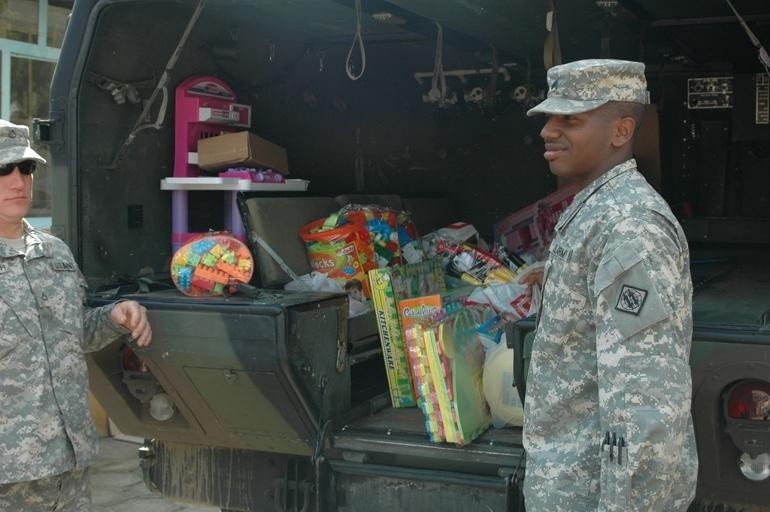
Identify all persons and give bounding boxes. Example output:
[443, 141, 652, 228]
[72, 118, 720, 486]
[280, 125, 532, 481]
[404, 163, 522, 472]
[0, 116, 153, 512]
[516, 56, 701, 511]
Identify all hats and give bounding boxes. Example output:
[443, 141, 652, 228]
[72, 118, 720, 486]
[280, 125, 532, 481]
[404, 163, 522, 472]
[526, 57, 651, 118]
[0, 121, 49, 167]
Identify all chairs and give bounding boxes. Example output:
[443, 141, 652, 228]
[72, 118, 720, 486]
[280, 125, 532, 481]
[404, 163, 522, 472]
[235, 189, 460, 366]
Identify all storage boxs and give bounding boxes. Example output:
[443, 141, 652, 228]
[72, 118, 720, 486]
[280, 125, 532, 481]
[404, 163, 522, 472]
[396, 283, 478, 400]
[367, 256, 450, 410]
[196, 128, 291, 179]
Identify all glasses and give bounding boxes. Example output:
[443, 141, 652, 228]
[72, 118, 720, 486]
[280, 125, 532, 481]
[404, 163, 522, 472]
[0, 158, 38, 177]
